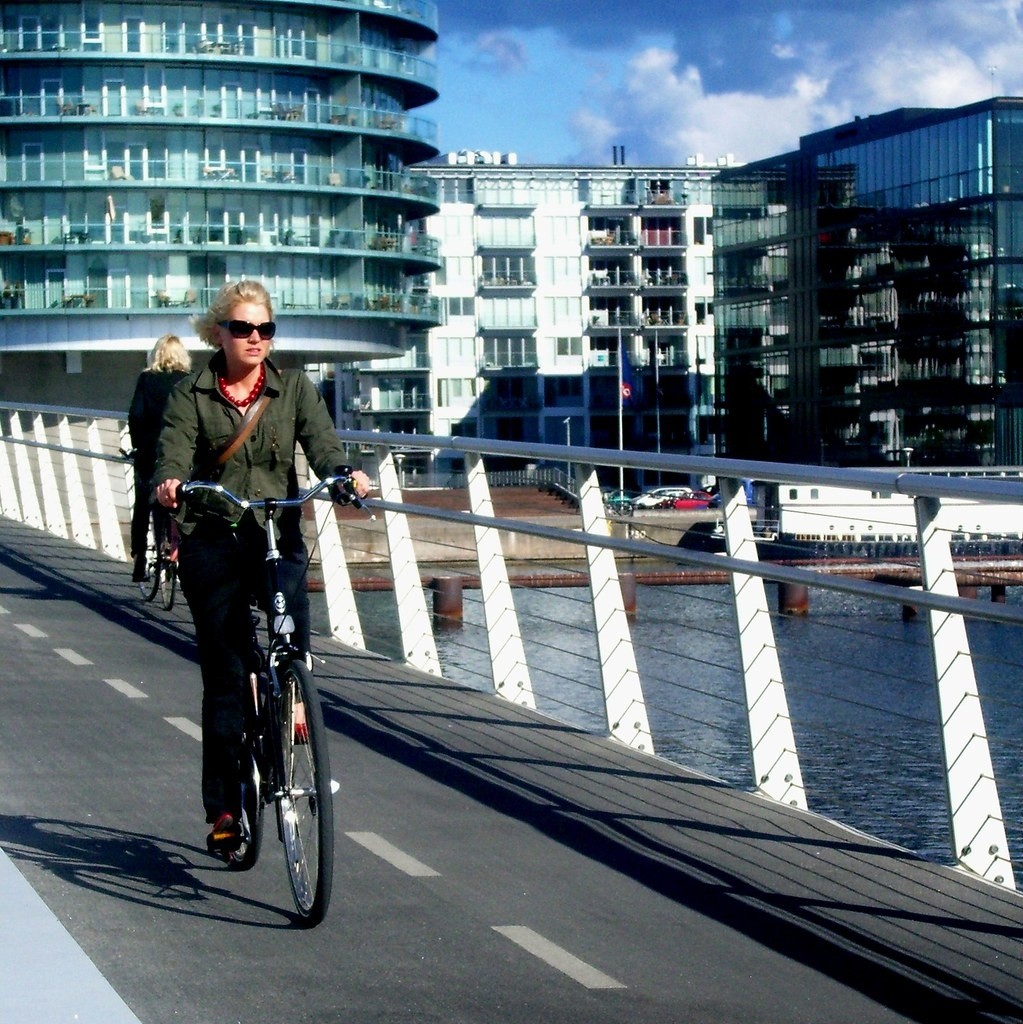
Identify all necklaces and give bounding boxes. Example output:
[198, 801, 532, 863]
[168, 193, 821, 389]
[217, 360, 265, 407]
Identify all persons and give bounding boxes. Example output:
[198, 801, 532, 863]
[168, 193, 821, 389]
[127, 334, 193, 581]
[152, 279, 371, 863]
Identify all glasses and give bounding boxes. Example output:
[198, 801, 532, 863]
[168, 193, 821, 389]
[217, 320, 275, 340]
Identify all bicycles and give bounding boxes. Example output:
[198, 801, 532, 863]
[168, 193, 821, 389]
[119, 447, 178, 611]
[148, 464, 379, 929]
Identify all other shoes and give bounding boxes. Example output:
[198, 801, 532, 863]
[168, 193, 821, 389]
[292, 721, 311, 741]
[206, 813, 239, 848]
[132, 553, 147, 579]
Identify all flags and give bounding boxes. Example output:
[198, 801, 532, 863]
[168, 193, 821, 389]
[621, 342, 641, 409]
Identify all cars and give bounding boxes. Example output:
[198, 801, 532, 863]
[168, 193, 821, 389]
[601, 484, 753, 510]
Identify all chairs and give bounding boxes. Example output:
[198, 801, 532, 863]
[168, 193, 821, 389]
[0, 37, 436, 314]
